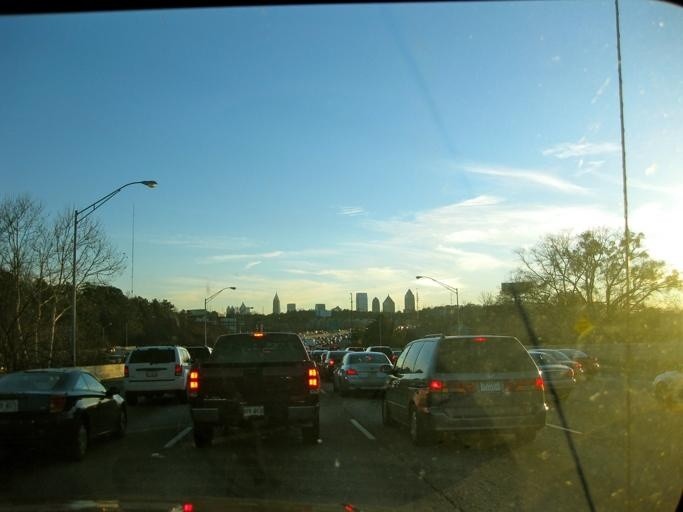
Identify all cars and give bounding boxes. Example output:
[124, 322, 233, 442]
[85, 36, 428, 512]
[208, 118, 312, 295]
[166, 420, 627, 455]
[332, 352, 394, 397]
[297, 324, 416, 382]
[554, 348, 600, 381]
[526, 349, 584, 387]
[183, 346, 213, 362]
[652, 358, 682, 409]
[122, 345, 193, 406]
[527, 352, 576, 403]
[0, 367, 127, 462]
[107, 347, 115, 352]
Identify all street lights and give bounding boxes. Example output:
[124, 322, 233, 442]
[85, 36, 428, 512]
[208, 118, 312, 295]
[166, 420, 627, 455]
[349, 293, 352, 335]
[95, 323, 111, 348]
[236, 307, 253, 334]
[72, 181, 157, 367]
[415, 275, 460, 336]
[204, 287, 236, 346]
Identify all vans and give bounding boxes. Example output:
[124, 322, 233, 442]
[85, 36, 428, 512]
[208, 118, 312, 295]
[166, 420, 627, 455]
[379, 332, 547, 446]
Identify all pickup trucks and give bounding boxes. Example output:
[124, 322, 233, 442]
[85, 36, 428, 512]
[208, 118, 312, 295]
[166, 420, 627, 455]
[186, 331, 322, 449]
